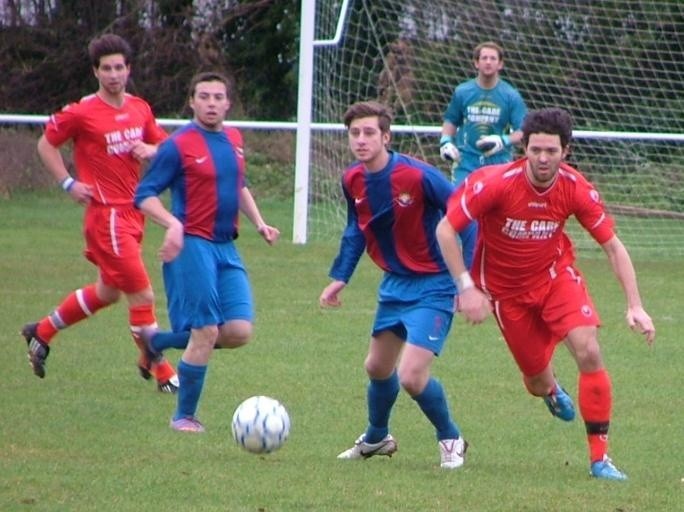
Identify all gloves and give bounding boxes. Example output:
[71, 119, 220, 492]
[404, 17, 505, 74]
[476, 134, 505, 159]
[439, 141, 462, 165]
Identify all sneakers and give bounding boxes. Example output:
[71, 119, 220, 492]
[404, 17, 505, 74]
[22, 322, 50, 379]
[337, 433, 397, 460]
[157, 374, 179, 392]
[138, 327, 165, 380]
[170, 415, 204, 432]
[439, 433, 465, 469]
[589, 454, 627, 481]
[541, 385, 576, 421]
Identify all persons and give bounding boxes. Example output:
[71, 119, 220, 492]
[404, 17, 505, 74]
[129, 71, 281, 435]
[21, 32, 180, 396]
[314, 99, 475, 472]
[437, 40, 529, 267]
[431, 107, 657, 482]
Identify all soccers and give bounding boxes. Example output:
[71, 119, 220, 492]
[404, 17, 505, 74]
[232, 395, 290, 454]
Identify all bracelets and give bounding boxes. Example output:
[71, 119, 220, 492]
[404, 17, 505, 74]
[57, 175, 74, 192]
[453, 269, 475, 292]
